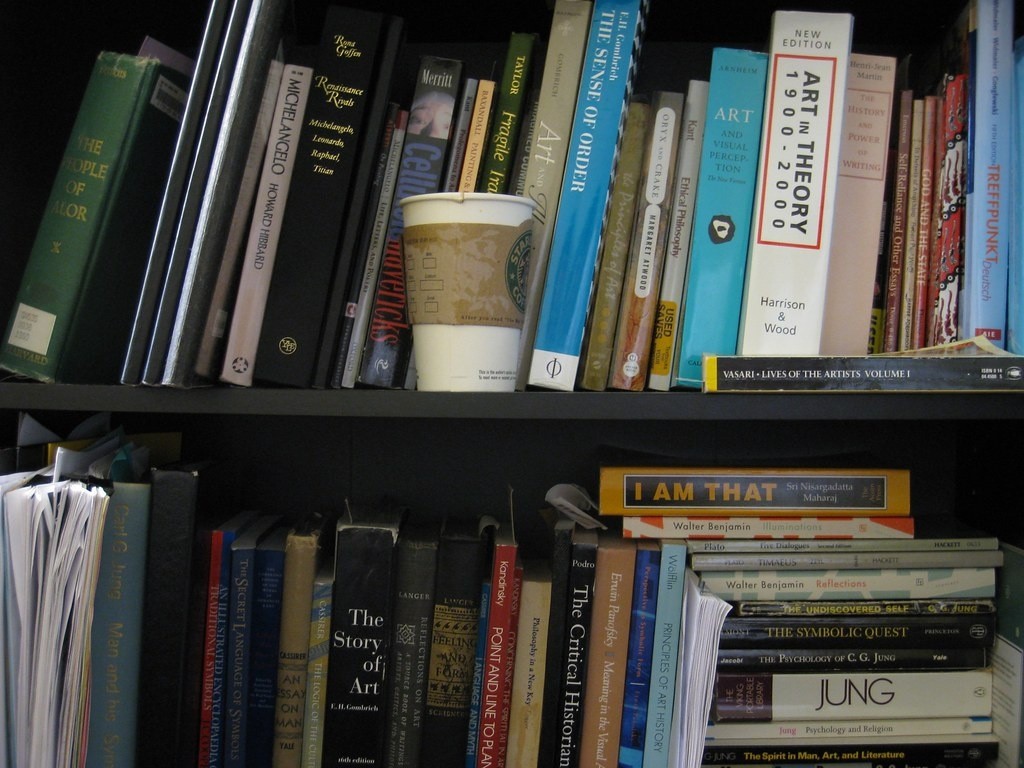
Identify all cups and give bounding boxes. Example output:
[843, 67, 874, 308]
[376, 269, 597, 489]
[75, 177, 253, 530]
[399, 192, 537, 392]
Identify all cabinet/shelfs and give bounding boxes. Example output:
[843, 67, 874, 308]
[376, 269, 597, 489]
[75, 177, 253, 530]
[1, 0, 1024, 768]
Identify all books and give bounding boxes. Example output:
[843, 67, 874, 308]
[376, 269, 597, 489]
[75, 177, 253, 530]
[0, 412, 1024, 768]
[1, 0, 1024, 392]
